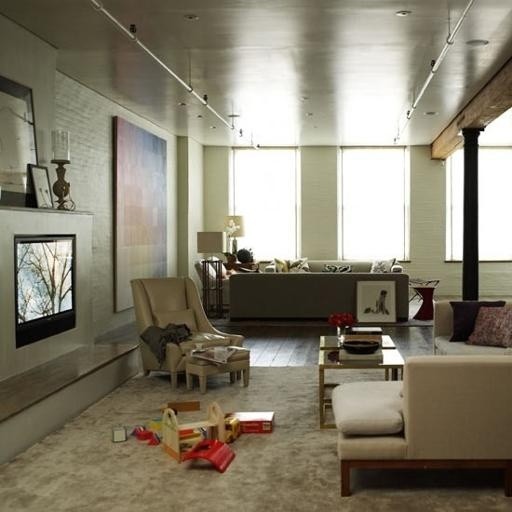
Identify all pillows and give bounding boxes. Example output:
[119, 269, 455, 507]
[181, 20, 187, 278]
[322, 263, 352, 273]
[151, 307, 199, 334]
[273, 256, 310, 273]
[466, 306, 512, 347]
[448, 301, 506, 342]
[369, 257, 396, 273]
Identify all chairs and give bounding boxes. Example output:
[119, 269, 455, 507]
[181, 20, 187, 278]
[129, 276, 246, 392]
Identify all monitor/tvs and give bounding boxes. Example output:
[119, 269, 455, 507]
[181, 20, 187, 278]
[14, 233, 77, 347]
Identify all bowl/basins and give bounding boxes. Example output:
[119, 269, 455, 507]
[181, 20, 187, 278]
[342, 339, 379, 354]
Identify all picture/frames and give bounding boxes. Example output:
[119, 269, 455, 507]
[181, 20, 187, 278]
[356, 280, 398, 324]
[27, 163, 54, 209]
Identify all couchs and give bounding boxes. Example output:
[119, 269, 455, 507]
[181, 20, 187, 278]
[263, 259, 403, 273]
[229, 273, 409, 322]
[193, 255, 238, 314]
[432, 299, 512, 355]
[331, 354, 512, 499]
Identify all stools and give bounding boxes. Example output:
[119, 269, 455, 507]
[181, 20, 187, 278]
[185, 344, 250, 395]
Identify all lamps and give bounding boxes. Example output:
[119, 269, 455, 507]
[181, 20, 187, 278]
[196, 231, 227, 318]
[223, 216, 246, 255]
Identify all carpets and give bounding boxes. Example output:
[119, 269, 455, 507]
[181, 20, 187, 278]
[0, 366, 512, 512]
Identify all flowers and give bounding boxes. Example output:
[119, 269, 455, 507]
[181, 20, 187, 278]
[326, 311, 355, 325]
[222, 219, 241, 237]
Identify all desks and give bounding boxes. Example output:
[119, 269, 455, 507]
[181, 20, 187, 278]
[223, 262, 260, 272]
[407, 280, 440, 321]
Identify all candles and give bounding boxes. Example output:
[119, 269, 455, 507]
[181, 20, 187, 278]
[50, 129, 69, 161]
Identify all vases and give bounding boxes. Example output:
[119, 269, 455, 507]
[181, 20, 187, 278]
[336, 324, 346, 348]
[225, 238, 234, 255]
